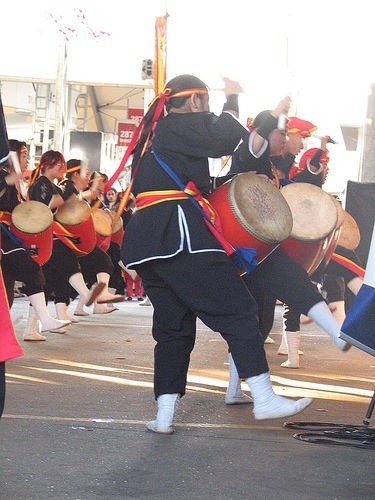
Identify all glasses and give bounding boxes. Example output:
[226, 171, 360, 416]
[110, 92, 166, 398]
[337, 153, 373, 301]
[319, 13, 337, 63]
[55, 163, 65, 167]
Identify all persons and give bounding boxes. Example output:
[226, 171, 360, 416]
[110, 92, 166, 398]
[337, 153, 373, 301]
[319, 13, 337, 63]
[0, 139, 151, 341]
[225, 95, 353, 404]
[0, 94, 24, 413]
[120, 75, 313, 434]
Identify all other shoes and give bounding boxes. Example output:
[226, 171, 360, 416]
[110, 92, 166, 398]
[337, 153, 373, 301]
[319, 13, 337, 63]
[139, 299, 152, 306]
[278, 345, 304, 355]
[127, 296, 144, 301]
[23, 301, 119, 341]
[335, 338, 352, 352]
[97, 294, 125, 304]
[224, 394, 254, 405]
[280, 359, 300, 369]
[253, 398, 312, 421]
[145, 420, 174, 434]
[264, 335, 274, 344]
[84, 282, 106, 306]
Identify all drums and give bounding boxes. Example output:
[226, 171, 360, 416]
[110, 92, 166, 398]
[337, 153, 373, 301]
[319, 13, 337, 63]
[277, 182, 361, 278]
[54, 198, 96, 256]
[204, 171, 294, 278]
[93, 209, 124, 253]
[10, 200, 53, 267]
